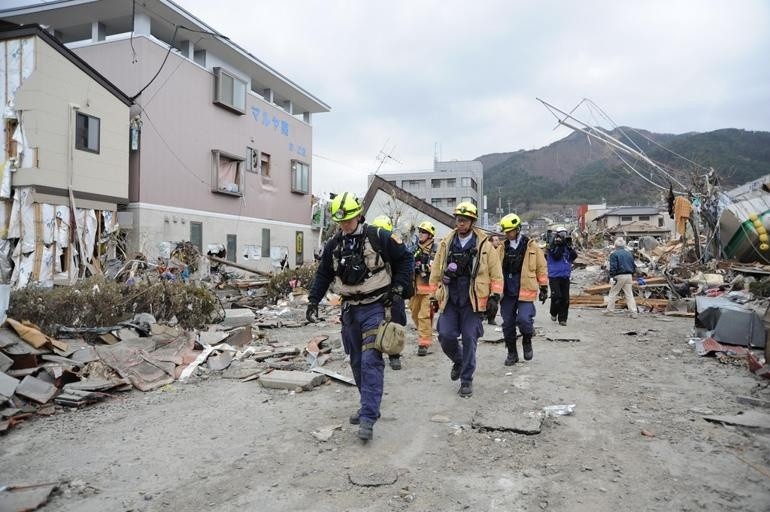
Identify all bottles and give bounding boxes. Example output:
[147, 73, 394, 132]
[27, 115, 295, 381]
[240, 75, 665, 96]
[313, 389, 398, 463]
[442, 262, 457, 286]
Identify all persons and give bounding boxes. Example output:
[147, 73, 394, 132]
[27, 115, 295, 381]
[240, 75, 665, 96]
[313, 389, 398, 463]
[373, 200, 550, 398]
[305, 191, 413, 440]
[281, 255, 289, 273]
[601, 236, 638, 318]
[547, 226, 578, 326]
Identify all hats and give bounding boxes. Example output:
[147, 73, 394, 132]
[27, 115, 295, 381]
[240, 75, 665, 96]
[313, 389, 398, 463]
[615, 237, 626, 246]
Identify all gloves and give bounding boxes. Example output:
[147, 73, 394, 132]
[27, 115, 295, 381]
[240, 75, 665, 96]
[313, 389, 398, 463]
[486, 293, 500, 318]
[381, 289, 399, 307]
[554, 237, 563, 245]
[429, 298, 439, 312]
[306, 298, 325, 323]
[539, 286, 547, 304]
[609, 277, 617, 285]
[415, 264, 428, 274]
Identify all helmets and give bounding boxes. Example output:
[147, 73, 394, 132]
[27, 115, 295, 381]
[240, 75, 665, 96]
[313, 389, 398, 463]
[373, 216, 392, 232]
[501, 213, 521, 233]
[418, 221, 436, 237]
[453, 202, 478, 220]
[331, 191, 364, 222]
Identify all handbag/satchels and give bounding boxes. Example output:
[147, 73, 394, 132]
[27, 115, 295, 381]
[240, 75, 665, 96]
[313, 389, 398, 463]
[375, 320, 406, 355]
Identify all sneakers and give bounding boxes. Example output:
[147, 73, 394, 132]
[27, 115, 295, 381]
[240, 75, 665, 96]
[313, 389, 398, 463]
[551, 315, 556, 320]
[602, 310, 614, 315]
[560, 320, 567, 326]
[358, 423, 373, 440]
[349, 412, 361, 425]
[451, 363, 462, 380]
[391, 358, 401, 370]
[488, 320, 496, 324]
[460, 383, 472, 396]
[627, 313, 638, 319]
[418, 346, 426, 356]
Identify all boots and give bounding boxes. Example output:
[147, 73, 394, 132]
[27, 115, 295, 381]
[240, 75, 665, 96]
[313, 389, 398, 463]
[523, 334, 533, 360]
[505, 340, 519, 365]
